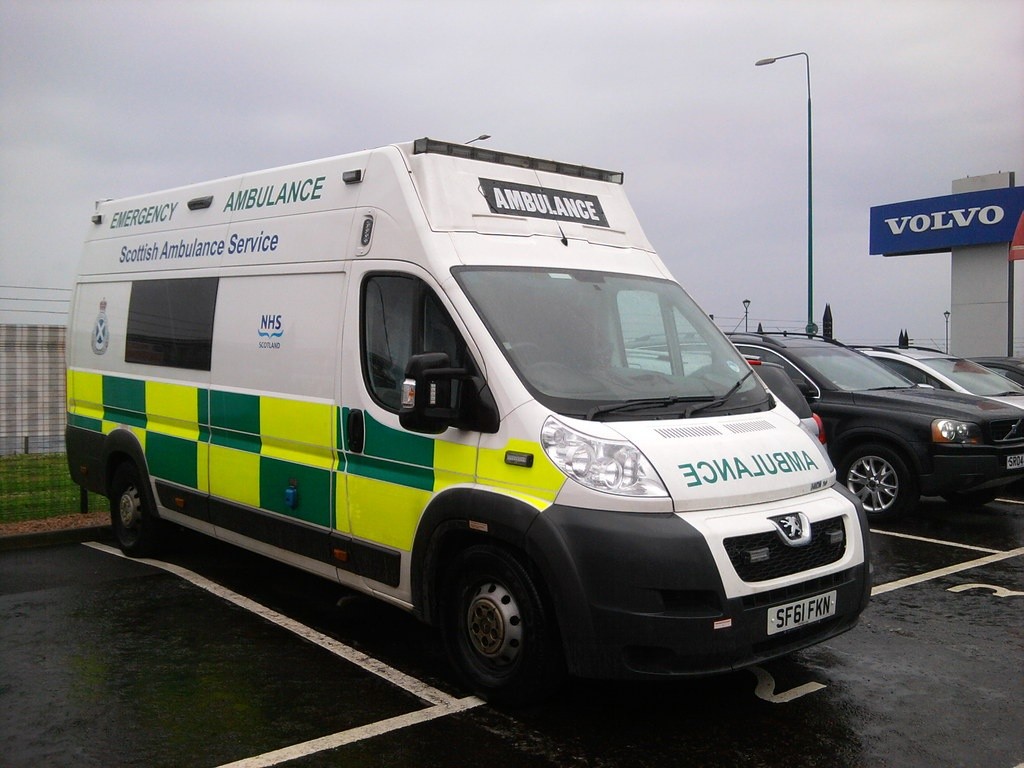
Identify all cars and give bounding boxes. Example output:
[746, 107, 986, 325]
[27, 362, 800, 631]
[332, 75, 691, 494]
[967, 356, 1024, 388]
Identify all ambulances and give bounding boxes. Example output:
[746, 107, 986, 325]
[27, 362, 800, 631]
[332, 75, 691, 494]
[66, 137, 873, 706]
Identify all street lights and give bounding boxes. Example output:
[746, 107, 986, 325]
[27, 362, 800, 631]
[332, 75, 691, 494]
[742, 298, 751, 332]
[944, 311, 951, 354]
[753, 51, 812, 340]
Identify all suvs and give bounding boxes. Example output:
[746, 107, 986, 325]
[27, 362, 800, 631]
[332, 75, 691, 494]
[846, 343, 1024, 411]
[624, 330, 1023, 528]
